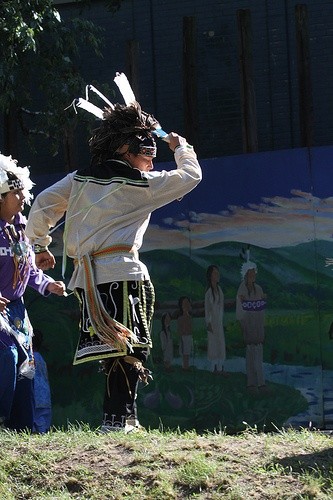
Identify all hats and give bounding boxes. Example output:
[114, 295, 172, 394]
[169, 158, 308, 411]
[0, 153, 36, 206]
[241, 250, 257, 280]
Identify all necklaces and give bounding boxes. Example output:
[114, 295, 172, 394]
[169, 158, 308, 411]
[3, 226, 27, 263]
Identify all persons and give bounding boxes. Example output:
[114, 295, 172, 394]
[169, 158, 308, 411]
[25, 102, 203, 433]
[0, 153, 66, 435]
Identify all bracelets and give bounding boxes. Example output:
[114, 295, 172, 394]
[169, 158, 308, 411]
[30, 243, 51, 254]
[173, 144, 195, 154]
